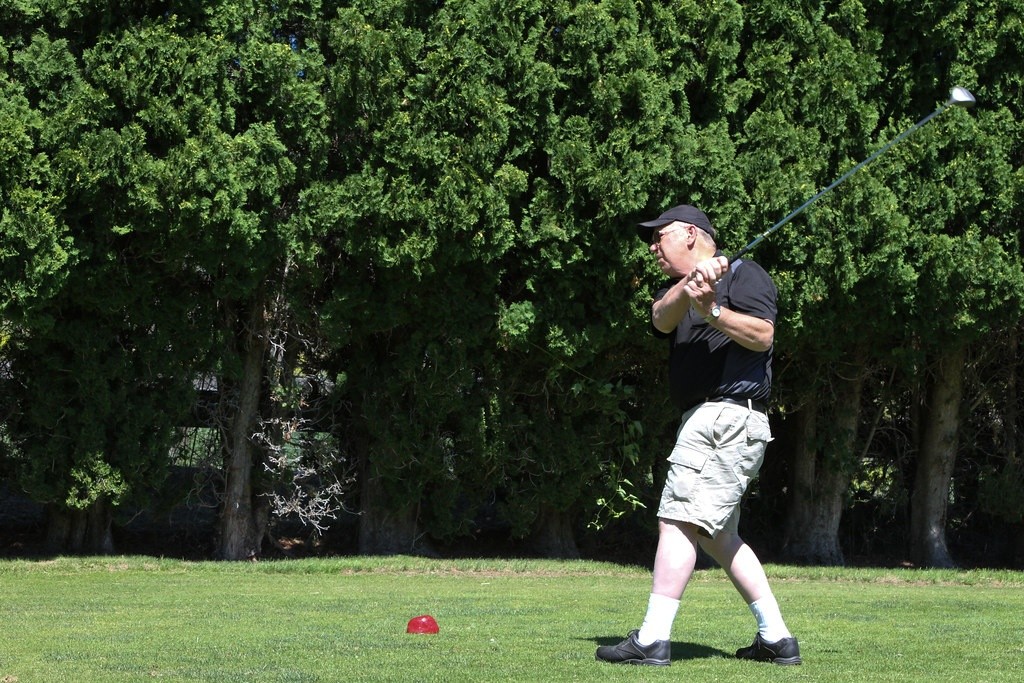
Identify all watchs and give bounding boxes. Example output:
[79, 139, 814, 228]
[704, 303, 721, 324]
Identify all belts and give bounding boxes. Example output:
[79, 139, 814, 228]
[707, 396, 766, 413]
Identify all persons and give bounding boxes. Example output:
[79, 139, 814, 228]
[596, 204, 802, 667]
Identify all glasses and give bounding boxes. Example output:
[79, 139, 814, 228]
[648, 229, 685, 245]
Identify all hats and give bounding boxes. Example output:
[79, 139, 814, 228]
[637, 205, 715, 243]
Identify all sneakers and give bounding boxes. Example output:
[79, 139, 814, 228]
[595, 629, 672, 667]
[735, 633, 802, 666]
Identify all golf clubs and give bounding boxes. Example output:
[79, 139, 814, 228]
[727, 85, 976, 267]
